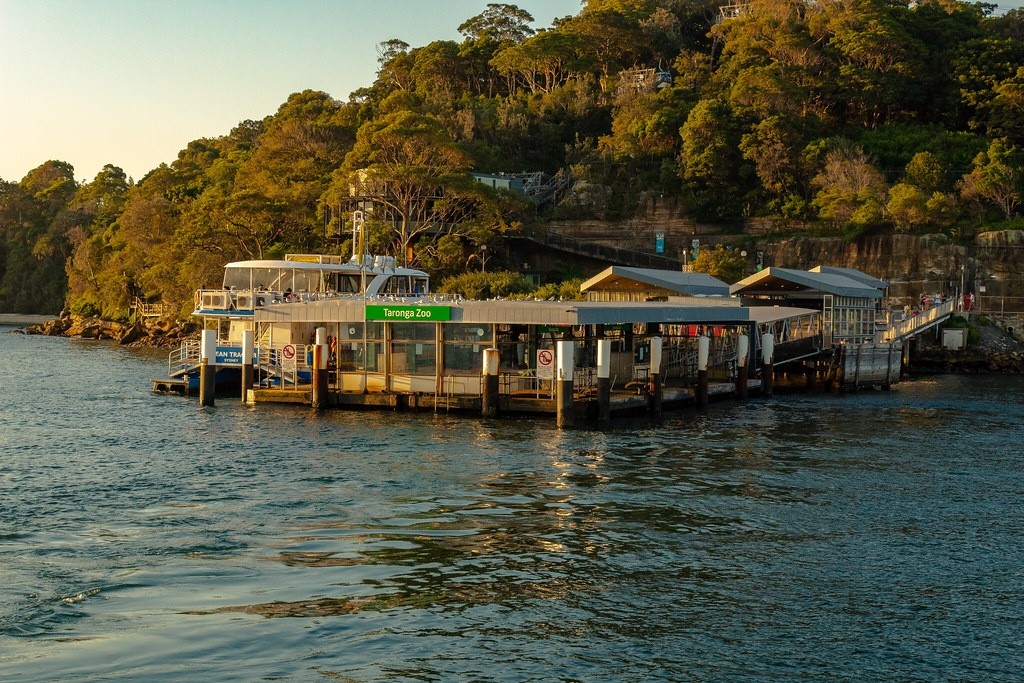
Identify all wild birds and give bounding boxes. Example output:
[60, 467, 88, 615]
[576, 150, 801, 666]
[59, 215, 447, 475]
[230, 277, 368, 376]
[260, 291, 567, 305]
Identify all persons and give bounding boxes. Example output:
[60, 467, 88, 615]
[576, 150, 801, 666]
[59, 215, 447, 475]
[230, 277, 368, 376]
[633, 319, 791, 343]
[885, 290, 978, 327]
[224, 278, 420, 306]
[307, 326, 337, 367]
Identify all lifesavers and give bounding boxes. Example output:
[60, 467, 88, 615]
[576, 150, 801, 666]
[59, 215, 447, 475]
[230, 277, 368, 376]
[285, 293, 301, 303]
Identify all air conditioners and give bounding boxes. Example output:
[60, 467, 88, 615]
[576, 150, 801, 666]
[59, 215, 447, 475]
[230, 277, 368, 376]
[202, 292, 230, 310]
[237, 293, 256, 312]
[255, 294, 274, 307]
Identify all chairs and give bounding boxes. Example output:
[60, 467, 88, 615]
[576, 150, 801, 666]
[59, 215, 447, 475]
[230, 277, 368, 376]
[284, 292, 564, 303]
